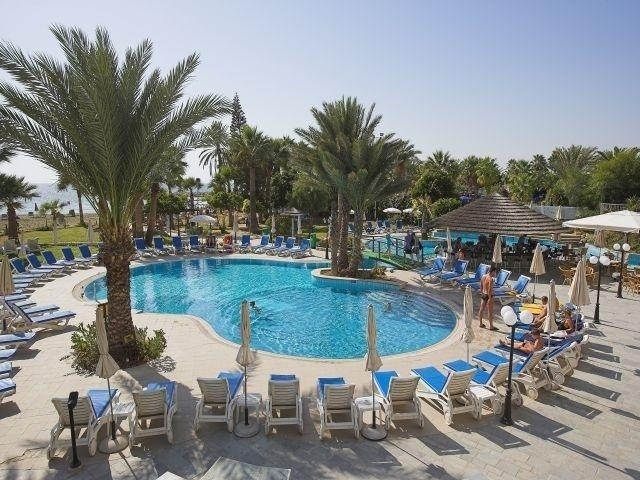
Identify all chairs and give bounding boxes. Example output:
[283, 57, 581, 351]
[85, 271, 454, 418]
[0, 297, 77, 395]
[129, 382, 179, 450]
[444, 302, 589, 413]
[49, 388, 119, 457]
[557, 248, 640, 297]
[317, 376, 360, 441]
[4, 244, 103, 295]
[265, 374, 304, 435]
[347, 219, 402, 235]
[373, 369, 424, 432]
[415, 256, 532, 314]
[411, 365, 481, 425]
[435, 240, 562, 273]
[198, 372, 245, 433]
[128, 234, 312, 261]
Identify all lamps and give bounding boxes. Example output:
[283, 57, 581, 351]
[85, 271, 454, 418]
[347, 219, 402, 235]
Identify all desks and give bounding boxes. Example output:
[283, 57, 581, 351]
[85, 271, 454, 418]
[613, 243, 632, 297]
[500, 305, 534, 427]
[355, 397, 382, 437]
[109, 400, 137, 439]
[590, 252, 611, 324]
[236, 393, 263, 425]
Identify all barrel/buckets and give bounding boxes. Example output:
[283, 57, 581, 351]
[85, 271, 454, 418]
[490, 327, 499, 330]
[480, 324, 485, 327]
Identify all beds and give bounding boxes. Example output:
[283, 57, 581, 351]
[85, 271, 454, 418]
[271, 215, 277, 246]
[297, 215, 302, 246]
[364, 304, 383, 428]
[337, 209, 354, 215]
[189, 215, 217, 244]
[87, 220, 96, 242]
[235, 301, 256, 426]
[403, 208, 423, 214]
[0, 254, 16, 319]
[96, 306, 120, 440]
[233, 212, 238, 243]
[446, 209, 640, 368]
[383, 208, 402, 213]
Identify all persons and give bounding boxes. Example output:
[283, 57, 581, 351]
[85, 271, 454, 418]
[207, 233, 215, 248]
[249, 301, 257, 309]
[225, 236, 232, 249]
[385, 229, 527, 265]
